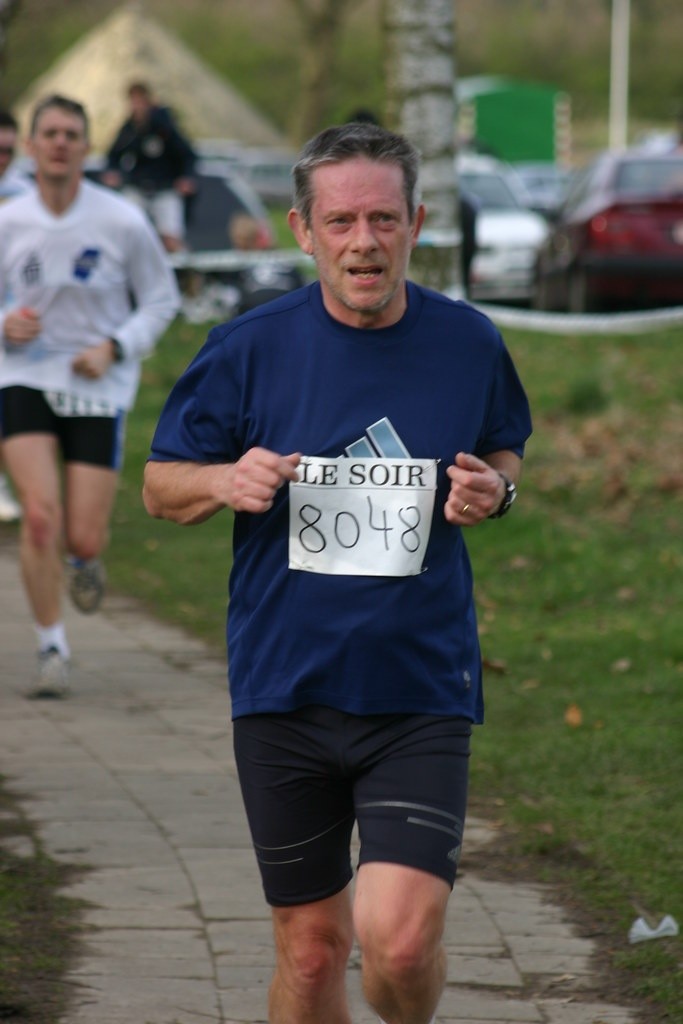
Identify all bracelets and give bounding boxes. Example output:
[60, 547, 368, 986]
[111, 340, 123, 361]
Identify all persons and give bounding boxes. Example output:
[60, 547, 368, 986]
[0, 107, 30, 208]
[99, 83, 204, 297]
[191, 216, 306, 317]
[0, 94, 180, 698]
[141, 123, 533, 1024]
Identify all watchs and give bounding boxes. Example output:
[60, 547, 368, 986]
[489, 473, 517, 519]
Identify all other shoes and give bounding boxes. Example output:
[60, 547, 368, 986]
[35, 647, 71, 696]
[71, 553, 102, 612]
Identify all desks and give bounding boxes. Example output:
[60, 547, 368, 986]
[171, 250, 313, 298]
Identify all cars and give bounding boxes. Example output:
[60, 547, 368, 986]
[16, 138, 302, 327]
[535, 150, 683, 316]
[457, 151, 551, 307]
[509, 159, 576, 222]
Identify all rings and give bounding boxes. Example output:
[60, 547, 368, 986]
[461, 504, 470, 514]
